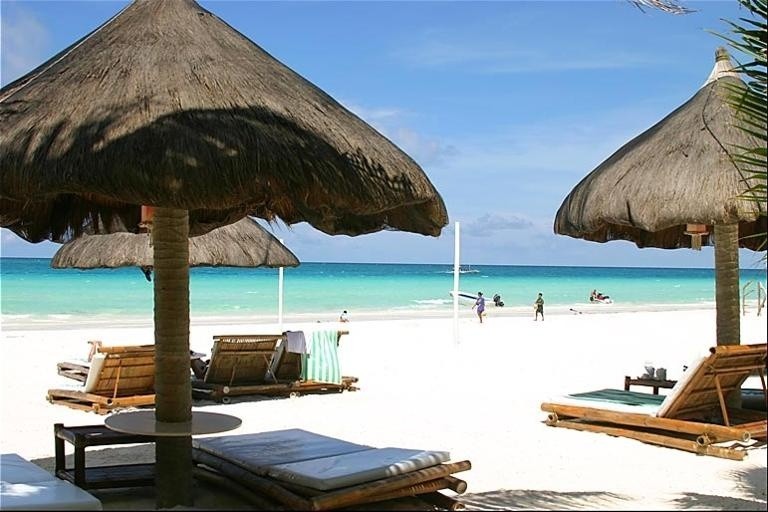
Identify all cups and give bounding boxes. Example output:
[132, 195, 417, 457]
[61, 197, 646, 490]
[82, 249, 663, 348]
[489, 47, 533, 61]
[656, 369, 666, 382]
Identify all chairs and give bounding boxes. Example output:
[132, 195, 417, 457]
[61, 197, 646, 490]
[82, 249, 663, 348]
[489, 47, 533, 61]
[45, 331, 358, 414]
[192, 429, 470, 511]
[1, 453, 102, 511]
[542, 341, 767, 461]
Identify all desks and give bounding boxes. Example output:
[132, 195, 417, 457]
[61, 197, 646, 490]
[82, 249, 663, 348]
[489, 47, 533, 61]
[53, 423, 157, 490]
[625, 375, 676, 396]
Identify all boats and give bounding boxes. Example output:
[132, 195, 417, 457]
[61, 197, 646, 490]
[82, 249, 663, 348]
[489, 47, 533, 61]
[449, 290, 502, 306]
[451, 264, 480, 274]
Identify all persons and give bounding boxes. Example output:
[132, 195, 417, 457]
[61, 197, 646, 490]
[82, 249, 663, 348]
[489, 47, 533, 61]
[471, 291, 487, 323]
[534, 293, 545, 321]
[591, 289, 598, 298]
[596, 292, 603, 299]
[340, 310, 348, 323]
